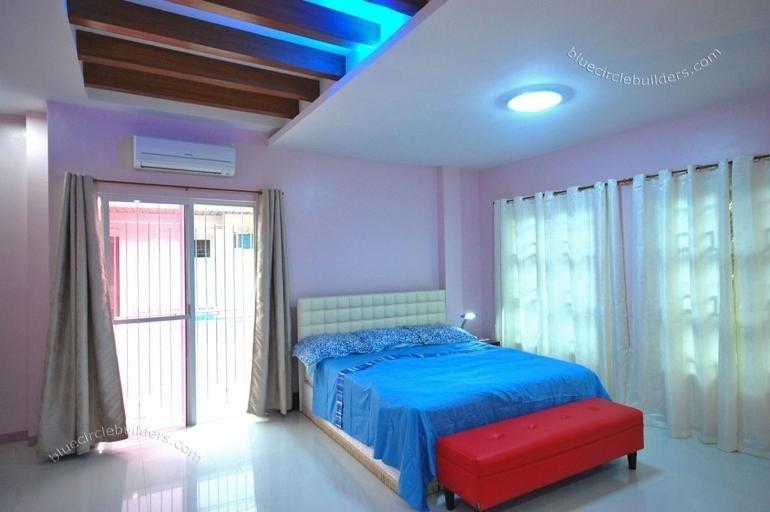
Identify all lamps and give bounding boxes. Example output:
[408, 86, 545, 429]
[496, 84, 574, 115]
[459, 312, 478, 329]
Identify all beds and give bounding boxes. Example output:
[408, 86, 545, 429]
[298, 289, 612, 511]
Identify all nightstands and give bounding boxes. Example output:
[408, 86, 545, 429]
[480, 337, 501, 346]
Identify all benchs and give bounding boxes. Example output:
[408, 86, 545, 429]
[436, 398, 644, 510]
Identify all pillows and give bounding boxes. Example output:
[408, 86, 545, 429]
[292, 332, 371, 375]
[403, 325, 479, 345]
[352, 326, 424, 354]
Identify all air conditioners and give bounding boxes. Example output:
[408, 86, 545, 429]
[133, 135, 237, 178]
